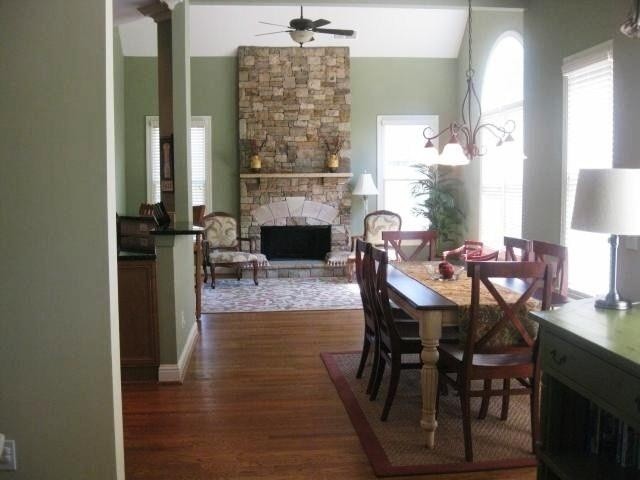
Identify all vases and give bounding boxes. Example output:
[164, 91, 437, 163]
[326, 153, 340, 172]
[250, 154, 262, 173]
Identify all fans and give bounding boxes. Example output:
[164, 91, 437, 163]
[255, 5, 354, 37]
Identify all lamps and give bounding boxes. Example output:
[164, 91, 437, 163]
[570, 168, 640, 309]
[290, 28, 315, 47]
[352, 169, 377, 219]
[416, 0, 527, 167]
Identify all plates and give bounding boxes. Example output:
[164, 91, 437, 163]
[422, 263, 464, 281]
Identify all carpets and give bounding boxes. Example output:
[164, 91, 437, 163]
[198, 278, 399, 313]
[320, 351, 543, 480]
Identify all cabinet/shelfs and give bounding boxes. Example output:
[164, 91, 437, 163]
[539, 328, 640, 480]
[117, 260, 159, 368]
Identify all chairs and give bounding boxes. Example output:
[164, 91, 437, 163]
[202, 211, 259, 289]
[378, 228, 439, 261]
[193, 205, 205, 318]
[530, 238, 571, 295]
[434, 259, 553, 460]
[502, 235, 530, 264]
[353, 237, 422, 421]
[350, 210, 403, 260]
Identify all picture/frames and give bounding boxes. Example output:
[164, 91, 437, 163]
[159, 137, 174, 193]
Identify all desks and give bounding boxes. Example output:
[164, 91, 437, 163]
[363, 258, 545, 455]
